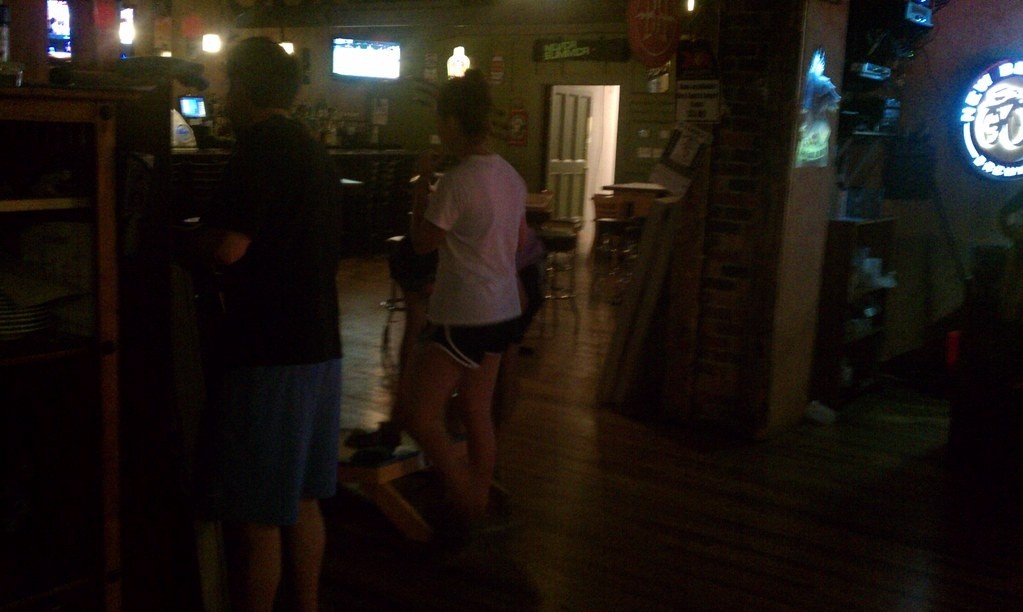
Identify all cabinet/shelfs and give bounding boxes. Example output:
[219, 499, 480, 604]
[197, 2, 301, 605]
[0, 86, 143, 612]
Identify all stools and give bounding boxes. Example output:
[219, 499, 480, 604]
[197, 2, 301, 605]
[381, 212, 638, 351]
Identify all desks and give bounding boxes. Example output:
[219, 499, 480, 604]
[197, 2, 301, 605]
[322, 147, 422, 248]
[167, 145, 237, 218]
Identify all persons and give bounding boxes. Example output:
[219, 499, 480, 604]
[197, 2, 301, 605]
[194, 36, 343, 612]
[400, 72, 547, 522]
